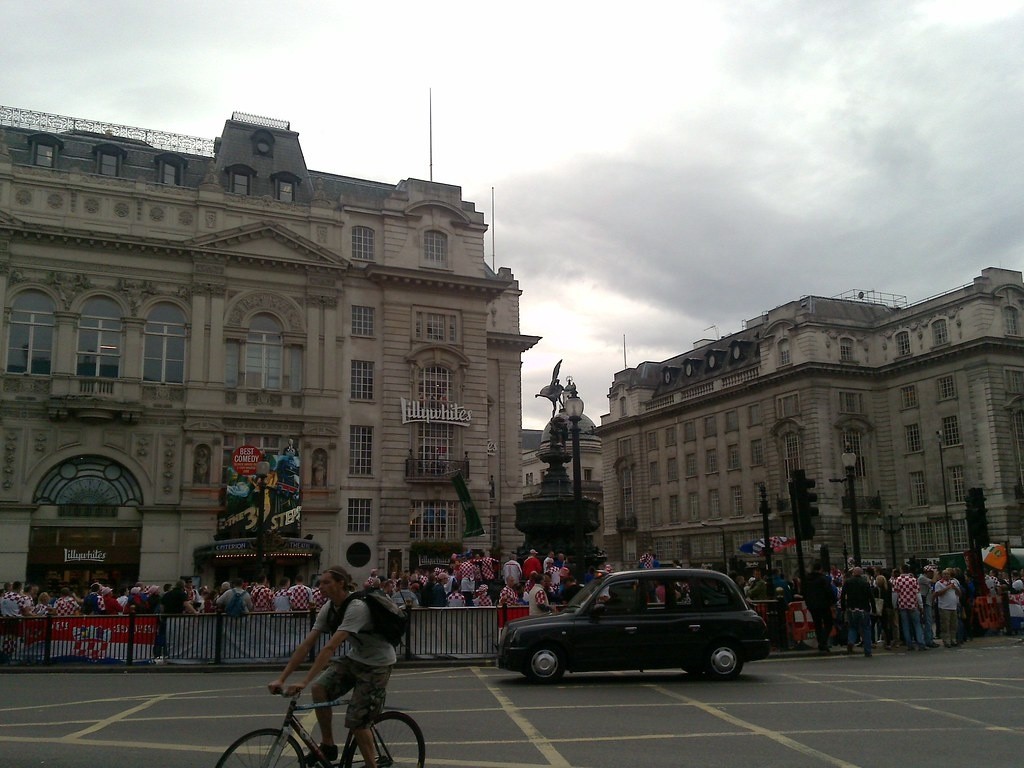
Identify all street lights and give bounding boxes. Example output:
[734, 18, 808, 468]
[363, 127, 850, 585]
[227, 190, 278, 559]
[843, 444, 864, 572]
[252, 454, 271, 584]
[563, 389, 586, 563]
[936, 429, 952, 552]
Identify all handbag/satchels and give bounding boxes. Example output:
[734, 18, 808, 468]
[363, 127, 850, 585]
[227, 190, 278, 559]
[399, 589, 412, 614]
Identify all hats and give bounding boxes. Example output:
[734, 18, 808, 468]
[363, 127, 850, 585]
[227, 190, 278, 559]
[131, 587, 141, 595]
[748, 577, 755, 584]
[149, 585, 160, 594]
[530, 549, 538, 554]
[144, 586, 152, 594]
[101, 586, 113, 597]
[476, 584, 489, 593]
[543, 575, 552, 582]
[135, 581, 143, 587]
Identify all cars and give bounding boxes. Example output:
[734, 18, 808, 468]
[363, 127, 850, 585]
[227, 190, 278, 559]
[495, 569, 771, 686]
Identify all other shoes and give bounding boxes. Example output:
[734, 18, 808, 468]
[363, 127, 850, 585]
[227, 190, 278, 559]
[298, 743, 339, 765]
[857, 640, 958, 652]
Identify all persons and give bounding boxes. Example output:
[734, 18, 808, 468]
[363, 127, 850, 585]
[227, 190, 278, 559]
[115, 576, 254, 666]
[251, 574, 328, 616]
[283, 438, 300, 457]
[451, 548, 580, 607]
[802, 557, 961, 658]
[196, 448, 208, 483]
[954, 566, 1024, 642]
[313, 454, 324, 486]
[269, 566, 397, 768]
[584, 546, 711, 605]
[742, 569, 805, 651]
[528, 574, 556, 616]
[0, 581, 123, 666]
[348, 566, 466, 607]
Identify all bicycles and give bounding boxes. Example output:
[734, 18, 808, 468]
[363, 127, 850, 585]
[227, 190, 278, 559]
[214, 682, 426, 768]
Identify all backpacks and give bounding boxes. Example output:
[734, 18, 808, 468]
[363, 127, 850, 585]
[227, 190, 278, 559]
[325, 588, 410, 648]
[225, 588, 247, 618]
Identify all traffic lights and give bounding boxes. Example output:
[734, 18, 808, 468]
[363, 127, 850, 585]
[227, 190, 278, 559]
[967, 488, 988, 539]
[788, 469, 820, 542]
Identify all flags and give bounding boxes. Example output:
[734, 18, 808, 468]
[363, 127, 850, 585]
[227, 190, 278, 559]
[739, 536, 796, 557]
